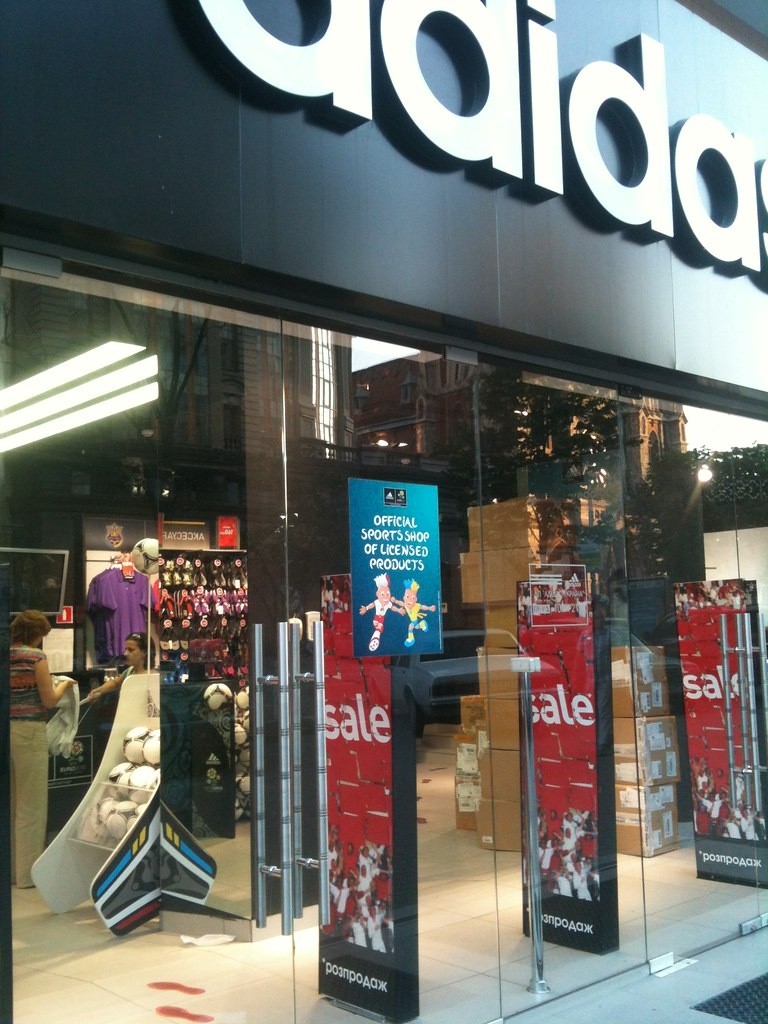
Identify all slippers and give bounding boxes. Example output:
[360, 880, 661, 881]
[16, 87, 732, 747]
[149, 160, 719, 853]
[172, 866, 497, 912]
[158, 557, 250, 683]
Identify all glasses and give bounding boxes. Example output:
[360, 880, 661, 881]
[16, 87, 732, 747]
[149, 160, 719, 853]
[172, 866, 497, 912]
[125, 633, 143, 643]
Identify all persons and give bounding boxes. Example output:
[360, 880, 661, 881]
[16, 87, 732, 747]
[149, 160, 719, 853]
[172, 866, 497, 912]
[88, 630, 159, 700]
[524, 799, 600, 904]
[322, 575, 352, 629]
[516, 581, 592, 629]
[674, 580, 747, 625]
[6, 610, 79, 891]
[691, 751, 768, 842]
[325, 820, 396, 954]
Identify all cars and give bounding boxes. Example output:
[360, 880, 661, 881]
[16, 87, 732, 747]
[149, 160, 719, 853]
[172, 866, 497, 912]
[392, 628, 529, 741]
[606, 610, 684, 706]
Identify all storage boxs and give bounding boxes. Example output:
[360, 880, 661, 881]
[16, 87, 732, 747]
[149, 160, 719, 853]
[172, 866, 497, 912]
[453, 496, 681, 859]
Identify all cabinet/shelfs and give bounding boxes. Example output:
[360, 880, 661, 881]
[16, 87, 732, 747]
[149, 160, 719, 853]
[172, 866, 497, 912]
[30, 672, 160, 939]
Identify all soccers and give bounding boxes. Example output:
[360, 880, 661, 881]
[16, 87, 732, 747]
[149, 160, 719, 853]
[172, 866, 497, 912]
[90, 726, 164, 840]
[131, 536, 160, 575]
[189, 683, 252, 820]
